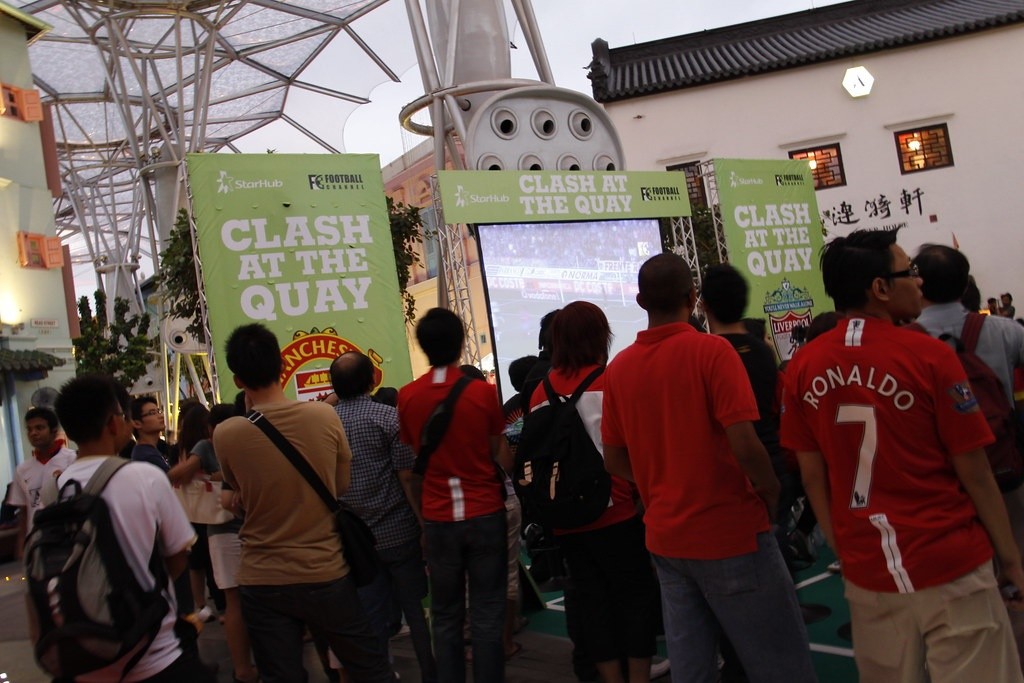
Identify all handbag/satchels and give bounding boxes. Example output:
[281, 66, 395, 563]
[172, 469, 235, 525]
[331, 497, 376, 565]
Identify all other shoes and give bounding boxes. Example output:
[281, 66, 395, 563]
[786, 529, 817, 564]
[649, 659, 671, 680]
[837, 621, 852, 640]
[232, 668, 262, 683]
[827, 560, 841, 572]
[178, 662, 220, 675]
[798, 603, 831, 624]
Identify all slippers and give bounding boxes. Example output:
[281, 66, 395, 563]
[512, 616, 529, 634]
[465, 646, 474, 664]
[505, 642, 522, 660]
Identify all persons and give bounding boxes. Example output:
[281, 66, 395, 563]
[212, 323, 398, 683]
[323, 351, 436, 683]
[5, 407, 77, 647]
[53, 376, 219, 683]
[398, 308, 514, 683]
[894, 244, 1024, 683]
[118, 392, 350, 683]
[501, 301, 671, 683]
[688, 264, 843, 683]
[780, 227, 1024, 683]
[602, 253, 819, 683]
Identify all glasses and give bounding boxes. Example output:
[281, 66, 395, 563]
[887, 262, 919, 279]
[139, 409, 163, 418]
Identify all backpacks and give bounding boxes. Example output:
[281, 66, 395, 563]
[512, 364, 611, 529]
[20, 455, 170, 678]
[908, 311, 1022, 481]
